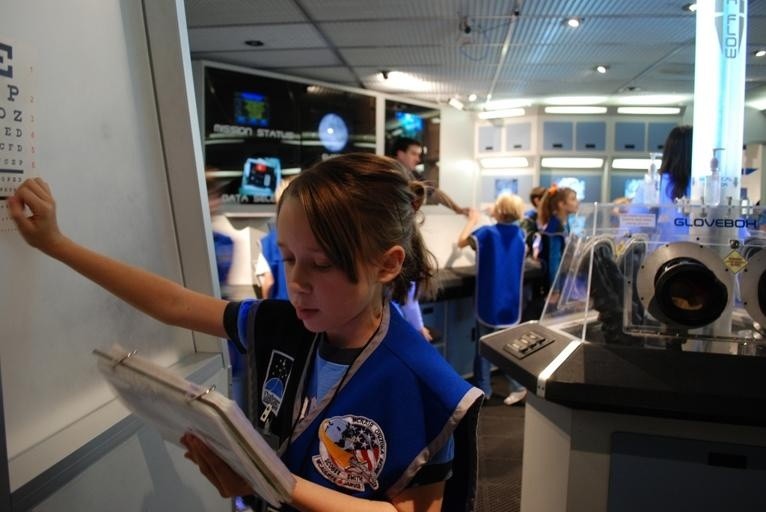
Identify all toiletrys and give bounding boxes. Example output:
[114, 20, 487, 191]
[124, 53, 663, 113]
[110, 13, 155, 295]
[643, 150, 663, 209]
[701, 147, 727, 207]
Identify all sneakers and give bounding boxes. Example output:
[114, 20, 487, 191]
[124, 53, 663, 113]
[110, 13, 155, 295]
[503, 389, 528, 406]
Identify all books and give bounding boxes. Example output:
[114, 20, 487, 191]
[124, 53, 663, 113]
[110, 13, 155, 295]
[98, 344, 296, 509]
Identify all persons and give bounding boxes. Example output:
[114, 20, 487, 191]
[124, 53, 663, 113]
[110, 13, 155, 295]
[386, 138, 472, 217]
[9, 153, 486, 512]
[522, 186, 587, 315]
[205, 169, 288, 303]
[445, 195, 527, 405]
[613, 124, 752, 306]
